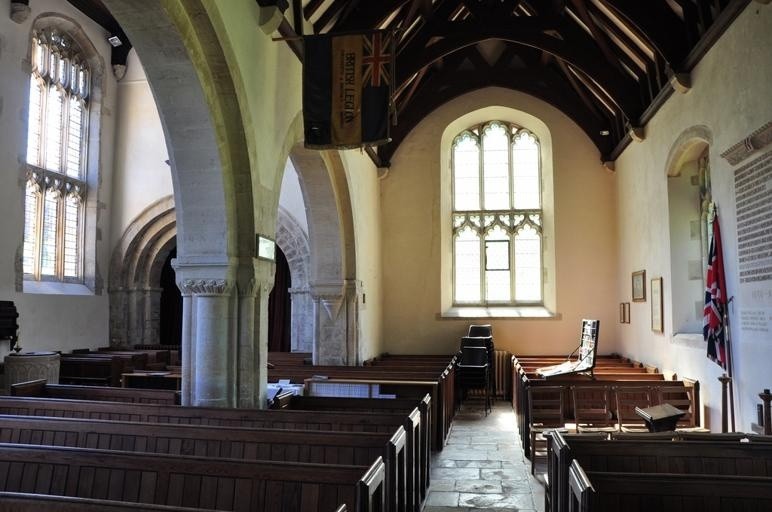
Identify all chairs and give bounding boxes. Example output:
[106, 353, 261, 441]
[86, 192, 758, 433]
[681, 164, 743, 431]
[455, 324, 496, 416]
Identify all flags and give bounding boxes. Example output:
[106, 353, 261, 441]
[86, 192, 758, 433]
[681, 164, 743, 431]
[301, 29, 395, 150]
[703, 204, 729, 371]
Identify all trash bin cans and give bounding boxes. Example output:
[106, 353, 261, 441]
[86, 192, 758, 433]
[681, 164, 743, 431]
[5, 351, 60, 395]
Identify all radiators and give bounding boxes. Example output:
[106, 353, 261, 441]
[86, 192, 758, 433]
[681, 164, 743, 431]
[466, 350, 511, 401]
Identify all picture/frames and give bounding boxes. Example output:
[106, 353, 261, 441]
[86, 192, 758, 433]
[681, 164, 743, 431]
[620, 269, 664, 335]
